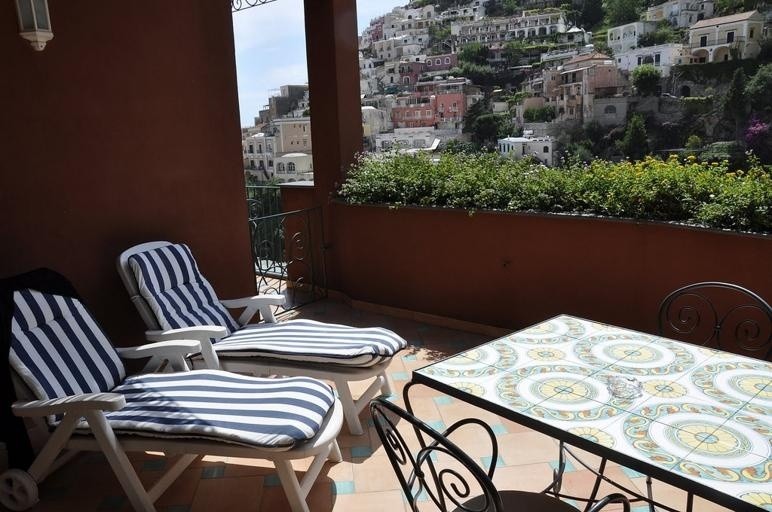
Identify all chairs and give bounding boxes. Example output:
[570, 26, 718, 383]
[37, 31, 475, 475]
[371, 396, 578, 509]
[0, 270, 343, 512]
[116, 240, 408, 435]
[654, 281, 772, 364]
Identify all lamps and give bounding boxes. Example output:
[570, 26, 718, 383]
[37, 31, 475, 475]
[15, 0, 54, 50]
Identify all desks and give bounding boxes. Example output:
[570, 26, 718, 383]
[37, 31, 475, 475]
[410, 312, 772, 509]
